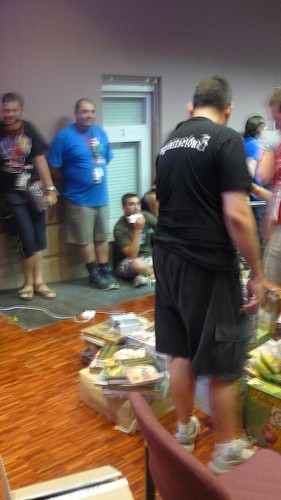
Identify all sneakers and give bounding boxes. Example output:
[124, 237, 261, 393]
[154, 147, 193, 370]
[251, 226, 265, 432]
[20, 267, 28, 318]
[89, 271, 120, 290]
[174, 416, 200, 454]
[135, 275, 156, 287]
[209, 450, 255, 475]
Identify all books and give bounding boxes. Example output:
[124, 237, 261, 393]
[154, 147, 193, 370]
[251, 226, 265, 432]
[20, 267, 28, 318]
[244, 309, 281, 402]
[80, 311, 150, 347]
[88, 342, 171, 402]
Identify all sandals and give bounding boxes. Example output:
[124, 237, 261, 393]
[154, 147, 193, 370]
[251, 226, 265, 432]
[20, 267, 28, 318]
[17, 282, 57, 301]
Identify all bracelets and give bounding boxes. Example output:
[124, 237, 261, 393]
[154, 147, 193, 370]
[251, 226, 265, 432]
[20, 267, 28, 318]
[44, 185, 56, 192]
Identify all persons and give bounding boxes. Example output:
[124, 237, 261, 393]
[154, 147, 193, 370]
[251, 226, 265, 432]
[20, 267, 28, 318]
[0, 92, 59, 302]
[257, 88, 281, 317]
[153, 75, 265, 475]
[47, 98, 120, 290]
[140, 180, 158, 217]
[241, 116, 272, 259]
[113, 193, 158, 287]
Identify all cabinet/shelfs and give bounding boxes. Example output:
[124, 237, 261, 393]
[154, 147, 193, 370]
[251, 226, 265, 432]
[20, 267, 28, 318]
[0, 198, 89, 291]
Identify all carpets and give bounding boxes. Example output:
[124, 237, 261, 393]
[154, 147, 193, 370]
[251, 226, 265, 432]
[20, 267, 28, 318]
[0, 273, 155, 330]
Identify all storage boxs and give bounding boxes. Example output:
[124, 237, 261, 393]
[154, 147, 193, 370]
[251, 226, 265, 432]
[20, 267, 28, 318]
[10, 465, 135, 500]
[79, 368, 174, 435]
[243, 376, 281, 451]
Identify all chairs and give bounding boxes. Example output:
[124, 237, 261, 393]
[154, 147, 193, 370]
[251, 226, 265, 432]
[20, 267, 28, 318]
[129, 390, 281, 500]
